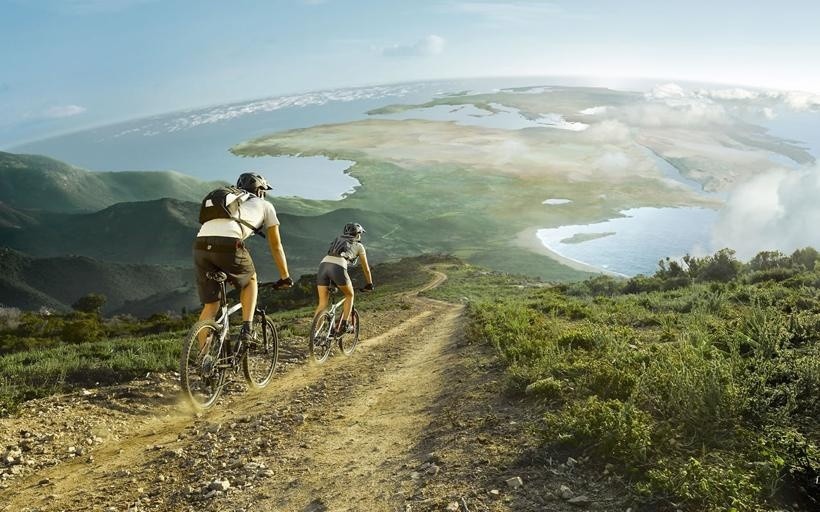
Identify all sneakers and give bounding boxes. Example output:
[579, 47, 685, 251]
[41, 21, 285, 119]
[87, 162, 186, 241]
[240, 328, 264, 346]
[341, 324, 354, 333]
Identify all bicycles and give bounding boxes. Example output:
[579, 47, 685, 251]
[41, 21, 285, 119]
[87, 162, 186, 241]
[309, 279, 369, 364]
[176, 270, 289, 413]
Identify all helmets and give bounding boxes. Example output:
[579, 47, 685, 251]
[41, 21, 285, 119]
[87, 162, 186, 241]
[237, 171, 272, 193]
[343, 222, 367, 236]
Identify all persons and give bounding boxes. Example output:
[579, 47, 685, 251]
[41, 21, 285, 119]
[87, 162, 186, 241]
[192, 172, 294, 372]
[308, 222, 374, 342]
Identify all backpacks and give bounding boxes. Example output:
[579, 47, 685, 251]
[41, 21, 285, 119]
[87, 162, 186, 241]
[327, 234, 356, 257]
[198, 185, 255, 225]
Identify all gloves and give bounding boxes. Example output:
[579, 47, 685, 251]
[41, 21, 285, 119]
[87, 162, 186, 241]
[365, 284, 375, 291]
[275, 278, 294, 290]
